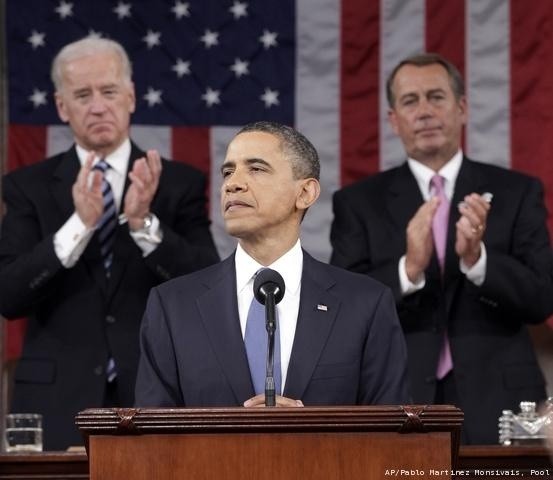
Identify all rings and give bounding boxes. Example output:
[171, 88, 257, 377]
[471, 226, 483, 235]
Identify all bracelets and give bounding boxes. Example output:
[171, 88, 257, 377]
[132, 216, 153, 235]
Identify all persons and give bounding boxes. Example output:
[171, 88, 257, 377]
[326, 53, 552, 446]
[132, 118, 415, 406]
[0, 36, 223, 453]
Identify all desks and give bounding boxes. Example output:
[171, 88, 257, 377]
[459, 444, 552, 479]
[0, 451, 89, 480]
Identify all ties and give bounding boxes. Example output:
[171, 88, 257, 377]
[94, 161, 119, 382]
[431, 175, 454, 381]
[244, 269, 281, 397]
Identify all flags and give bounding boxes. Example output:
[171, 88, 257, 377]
[1, 0, 550, 371]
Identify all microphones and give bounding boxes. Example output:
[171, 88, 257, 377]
[253, 268, 285, 332]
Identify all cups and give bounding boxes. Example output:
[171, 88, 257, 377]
[0, 412, 44, 456]
